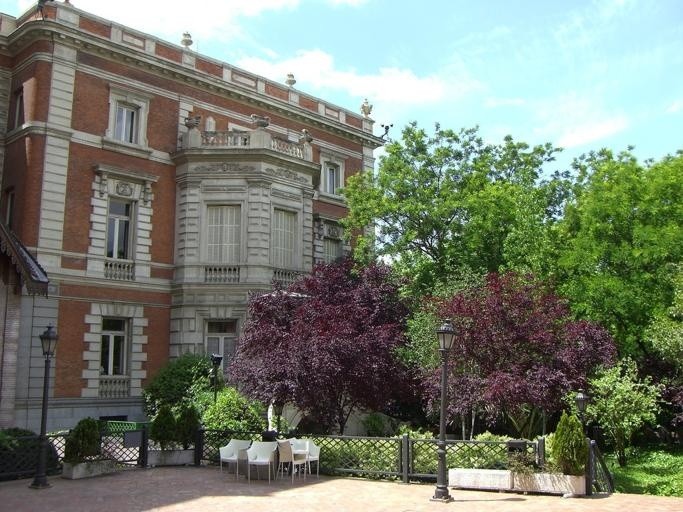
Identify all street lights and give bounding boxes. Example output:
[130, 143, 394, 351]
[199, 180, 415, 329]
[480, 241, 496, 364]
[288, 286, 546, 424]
[27, 320, 60, 490]
[428, 317, 461, 503]
[573, 388, 590, 435]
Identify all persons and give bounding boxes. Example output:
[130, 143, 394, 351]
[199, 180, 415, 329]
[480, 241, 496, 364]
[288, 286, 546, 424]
[267, 399, 275, 432]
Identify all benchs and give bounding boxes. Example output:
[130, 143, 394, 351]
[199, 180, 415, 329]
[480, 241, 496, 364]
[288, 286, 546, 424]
[447, 466, 586, 499]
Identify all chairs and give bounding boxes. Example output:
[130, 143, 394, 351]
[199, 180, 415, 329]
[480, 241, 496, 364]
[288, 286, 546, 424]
[217, 437, 321, 485]
